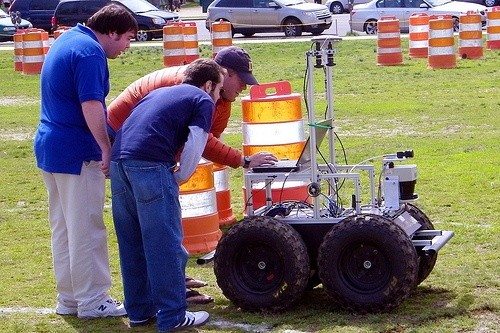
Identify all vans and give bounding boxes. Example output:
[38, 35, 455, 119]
[8, 0, 180, 42]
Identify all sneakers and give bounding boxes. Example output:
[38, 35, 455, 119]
[55, 302, 78, 315]
[130, 309, 161, 327]
[77, 295, 127, 318]
[172, 310, 210, 331]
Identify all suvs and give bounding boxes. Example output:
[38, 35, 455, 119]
[205, 0, 333, 37]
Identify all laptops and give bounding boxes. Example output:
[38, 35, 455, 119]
[252, 120, 333, 172]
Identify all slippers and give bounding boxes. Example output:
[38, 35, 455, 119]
[185, 287, 214, 305]
[185, 275, 208, 288]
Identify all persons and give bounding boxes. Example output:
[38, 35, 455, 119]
[33, 3, 138, 318]
[107, 46, 278, 303]
[110, 59, 226, 333]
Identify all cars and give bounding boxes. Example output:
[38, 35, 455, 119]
[324, 0, 500, 34]
[0, 8, 34, 41]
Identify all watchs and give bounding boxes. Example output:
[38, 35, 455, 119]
[243, 157, 251, 168]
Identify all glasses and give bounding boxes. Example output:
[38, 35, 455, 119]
[213, 82, 225, 97]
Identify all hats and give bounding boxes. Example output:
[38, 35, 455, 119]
[215, 46, 259, 85]
